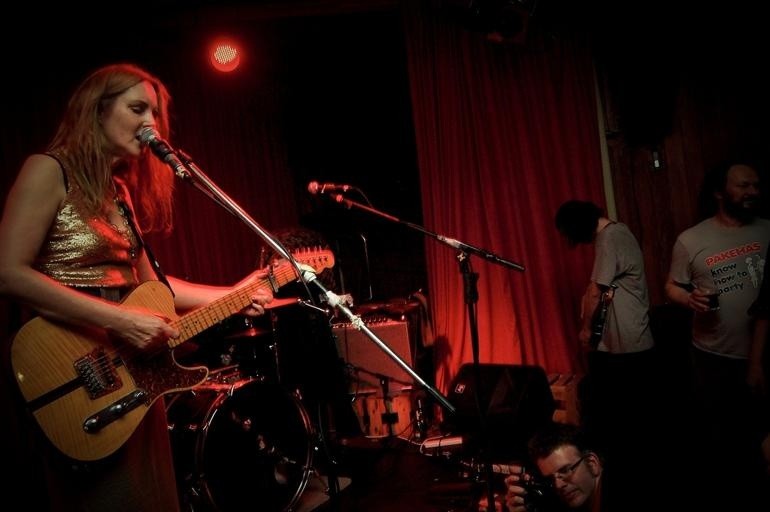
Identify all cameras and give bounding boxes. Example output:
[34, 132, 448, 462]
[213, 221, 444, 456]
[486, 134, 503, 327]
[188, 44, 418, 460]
[518, 476, 566, 512]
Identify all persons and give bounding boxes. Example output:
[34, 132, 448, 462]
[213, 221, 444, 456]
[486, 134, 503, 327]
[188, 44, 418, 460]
[254, 231, 363, 438]
[745, 239, 770, 512]
[477, 424, 653, 512]
[664, 157, 770, 508]
[554, 199, 654, 508]
[0, 61, 274, 512]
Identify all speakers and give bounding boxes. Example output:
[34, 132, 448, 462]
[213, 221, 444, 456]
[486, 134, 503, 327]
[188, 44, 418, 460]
[447, 363, 555, 448]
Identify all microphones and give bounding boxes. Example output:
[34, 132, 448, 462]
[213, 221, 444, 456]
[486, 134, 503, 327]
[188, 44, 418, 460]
[307, 180, 352, 194]
[140, 126, 192, 181]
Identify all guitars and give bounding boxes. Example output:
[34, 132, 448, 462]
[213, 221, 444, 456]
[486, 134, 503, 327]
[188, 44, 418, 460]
[10, 246, 335, 462]
[588, 288, 614, 346]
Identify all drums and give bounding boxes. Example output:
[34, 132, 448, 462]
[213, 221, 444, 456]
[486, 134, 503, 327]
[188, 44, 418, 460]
[165, 369, 314, 512]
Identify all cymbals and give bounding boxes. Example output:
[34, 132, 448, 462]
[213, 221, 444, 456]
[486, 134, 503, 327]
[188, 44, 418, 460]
[226, 327, 269, 340]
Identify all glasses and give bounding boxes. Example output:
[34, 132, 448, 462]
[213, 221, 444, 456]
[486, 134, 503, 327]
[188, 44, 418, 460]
[544, 455, 585, 490]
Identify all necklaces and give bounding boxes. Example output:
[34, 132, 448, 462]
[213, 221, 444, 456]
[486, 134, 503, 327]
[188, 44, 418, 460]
[105, 195, 139, 259]
